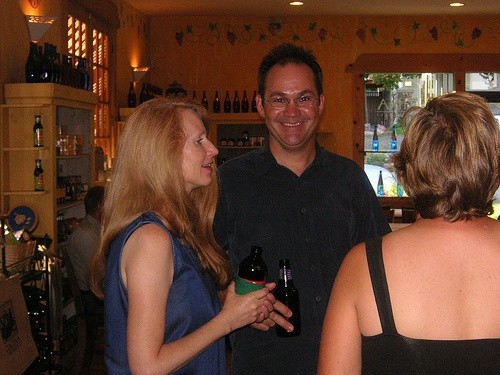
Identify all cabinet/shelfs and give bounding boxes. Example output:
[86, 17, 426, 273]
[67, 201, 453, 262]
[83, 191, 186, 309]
[0, 82, 99, 251]
[19, 255, 56, 375]
[206, 112, 266, 166]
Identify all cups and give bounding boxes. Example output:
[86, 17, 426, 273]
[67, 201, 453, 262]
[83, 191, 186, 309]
[220, 135, 265, 147]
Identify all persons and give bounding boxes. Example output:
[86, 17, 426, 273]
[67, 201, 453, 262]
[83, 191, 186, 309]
[67, 186, 104, 313]
[91, 99, 276, 375]
[317, 93, 500, 375]
[213, 43, 392, 375]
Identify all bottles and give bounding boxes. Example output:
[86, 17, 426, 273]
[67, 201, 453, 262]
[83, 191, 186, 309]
[201, 91, 208, 111]
[233, 90, 240, 113]
[213, 91, 220, 113]
[397, 181, 403, 197]
[242, 90, 249, 113]
[391, 125, 397, 149]
[376, 170, 384, 197]
[224, 90, 231, 112]
[25, 41, 90, 91]
[235, 245, 267, 296]
[34, 159, 45, 192]
[192, 90, 196, 101]
[127, 82, 136, 108]
[251, 90, 258, 113]
[57, 176, 66, 244]
[138, 83, 162, 105]
[274, 259, 301, 338]
[33, 115, 43, 147]
[372, 125, 379, 150]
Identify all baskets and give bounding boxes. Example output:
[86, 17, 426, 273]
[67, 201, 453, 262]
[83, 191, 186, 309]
[0, 241, 36, 271]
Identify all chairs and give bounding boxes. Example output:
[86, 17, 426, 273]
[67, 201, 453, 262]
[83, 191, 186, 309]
[62, 248, 106, 375]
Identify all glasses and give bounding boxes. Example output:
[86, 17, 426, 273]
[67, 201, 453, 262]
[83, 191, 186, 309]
[262, 95, 320, 109]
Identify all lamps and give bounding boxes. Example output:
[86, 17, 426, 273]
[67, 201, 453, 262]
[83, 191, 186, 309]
[133, 64, 151, 86]
[24, 15, 57, 43]
